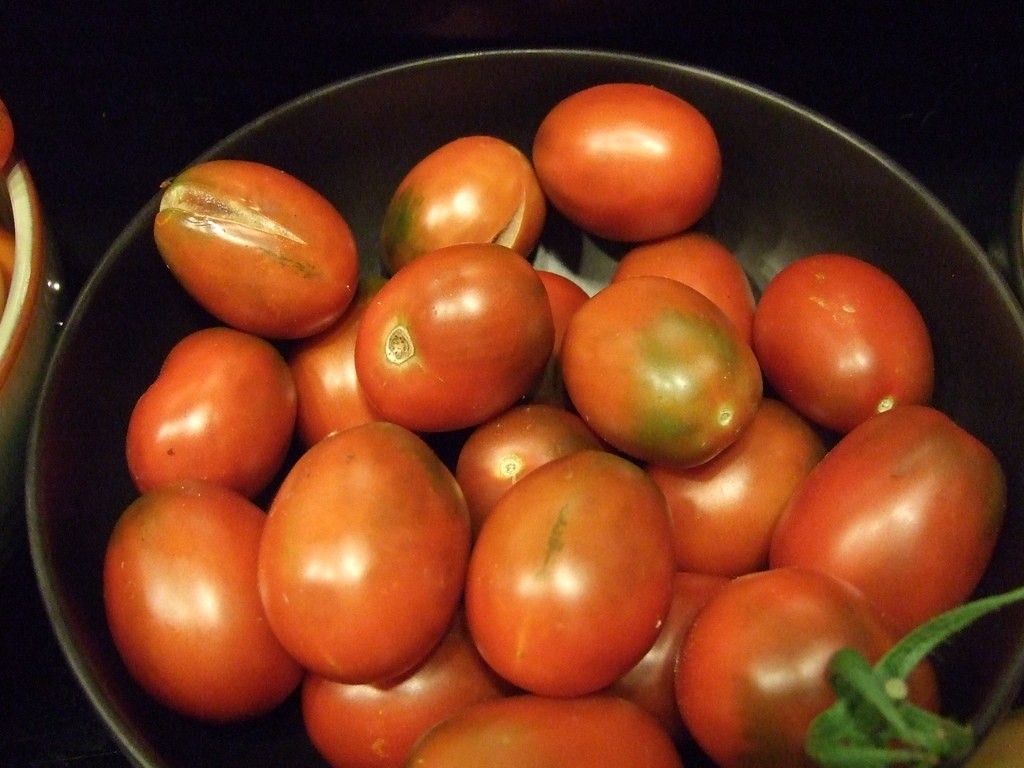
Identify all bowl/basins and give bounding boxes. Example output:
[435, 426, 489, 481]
[0, 162, 54, 431]
[23, 52, 1024, 768]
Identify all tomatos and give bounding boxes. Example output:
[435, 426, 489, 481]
[103, 83, 1005, 768]
